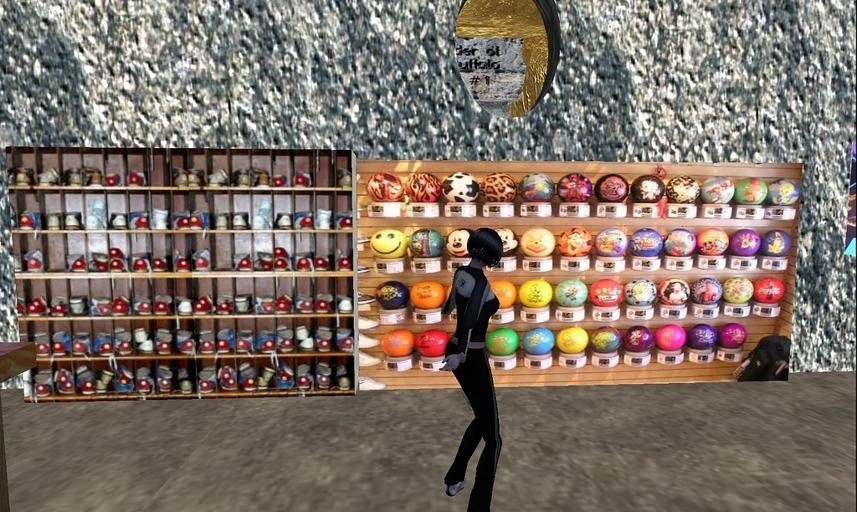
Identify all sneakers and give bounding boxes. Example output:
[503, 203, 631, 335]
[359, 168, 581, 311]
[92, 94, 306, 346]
[18, 208, 353, 230]
[34, 324, 355, 358]
[8, 166, 146, 189]
[67, 247, 211, 272]
[234, 248, 353, 273]
[34, 367, 355, 394]
[28, 296, 355, 316]
[174, 166, 315, 188]
[22, 251, 44, 274]
